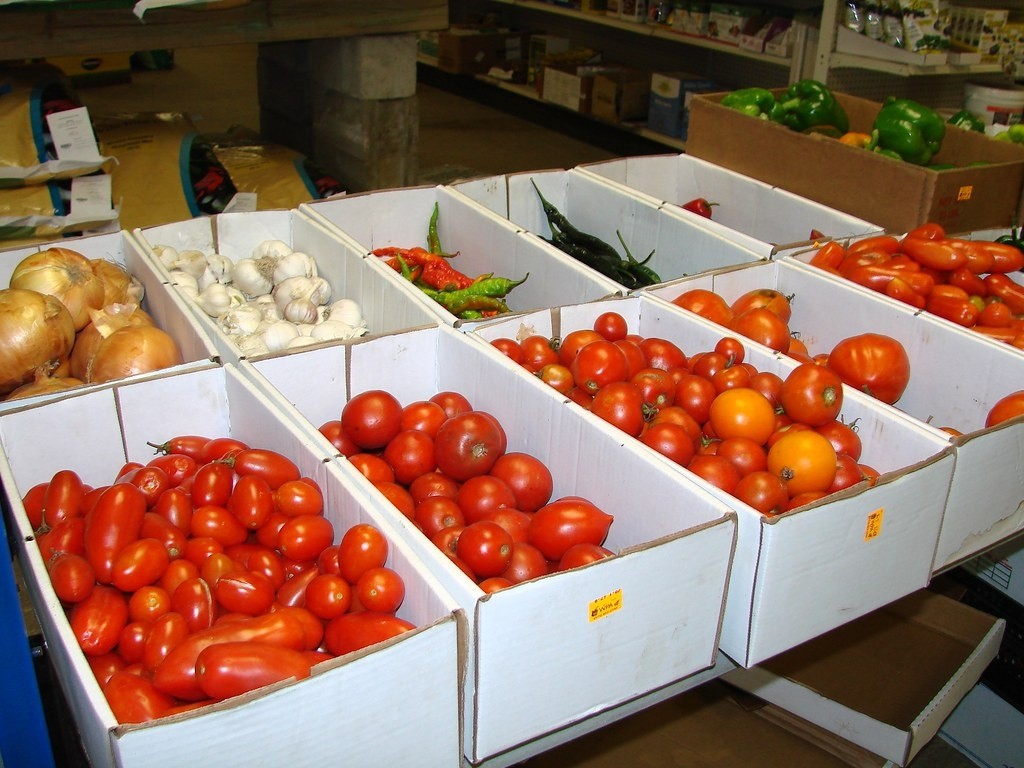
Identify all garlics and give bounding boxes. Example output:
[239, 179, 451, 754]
[152, 239, 368, 356]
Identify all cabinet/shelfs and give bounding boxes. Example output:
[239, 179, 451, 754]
[416, 0, 1004, 153]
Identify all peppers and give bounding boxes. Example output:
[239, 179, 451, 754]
[680, 198, 721, 220]
[528, 178, 663, 293]
[718, 79, 1024, 170]
[367, 202, 530, 319]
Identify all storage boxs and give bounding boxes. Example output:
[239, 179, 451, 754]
[571, 0, 608, 15]
[438, 23, 528, 74]
[507, 537, 1024, 768]
[0, 229, 222, 411]
[836, 6, 1024, 80]
[682, 89, 729, 142]
[590, 72, 652, 126]
[132, 207, 447, 369]
[465, 294, 955, 671]
[574, 153, 885, 263]
[640, 259, 1024, 578]
[300, 183, 623, 333]
[671, 8, 796, 59]
[647, 71, 713, 138]
[783, 227, 1024, 356]
[0, 362, 470, 768]
[528, 34, 569, 87]
[541, 62, 640, 114]
[685, 88, 1024, 235]
[237, 325, 738, 768]
[444, 168, 767, 296]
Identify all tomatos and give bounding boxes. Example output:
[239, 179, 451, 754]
[23, 436, 418, 725]
[315, 390, 619, 594]
[487, 224, 1024, 520]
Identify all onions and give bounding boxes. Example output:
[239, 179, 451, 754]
[0, 246, 186, 400]
